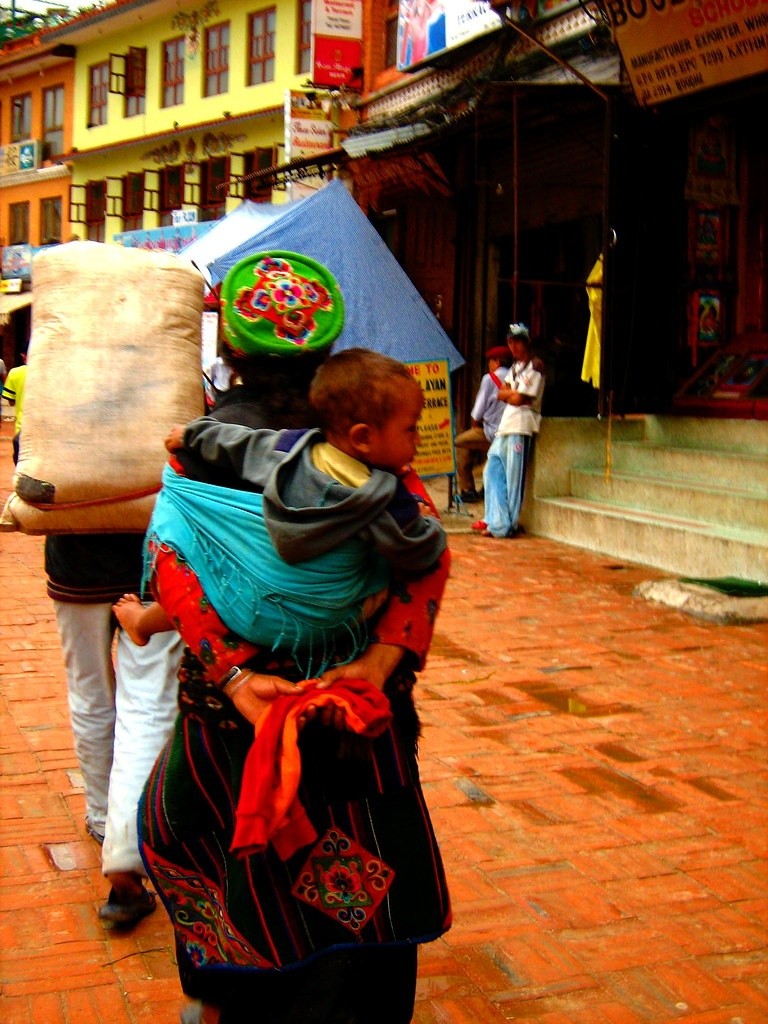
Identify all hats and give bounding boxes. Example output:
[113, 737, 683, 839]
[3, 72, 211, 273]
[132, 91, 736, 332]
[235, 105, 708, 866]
[506, 322, 529, 339]
[485, 347, 510, 362]
[218, 249, 345, 362]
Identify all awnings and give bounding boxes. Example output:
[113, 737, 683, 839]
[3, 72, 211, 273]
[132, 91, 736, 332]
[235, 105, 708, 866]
[218, 95, 477, 197]
[0, 291, 32, 326]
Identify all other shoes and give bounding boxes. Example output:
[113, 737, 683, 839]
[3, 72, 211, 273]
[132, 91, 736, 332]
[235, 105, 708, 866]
[481, 530, 491, 537]
[471, 520, 487, 531]
[452, 489, 478, 503]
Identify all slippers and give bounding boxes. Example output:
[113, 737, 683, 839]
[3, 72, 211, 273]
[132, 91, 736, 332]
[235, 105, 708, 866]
[85, 816, 105, 846]
[98, 877, 156, 921]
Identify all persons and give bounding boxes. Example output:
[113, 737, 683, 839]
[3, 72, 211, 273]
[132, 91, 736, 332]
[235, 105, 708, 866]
[13, 434, 189, 920]
[470, 323, 546, 536]
[454, 346, 511, 503]
[112, 249, 455, 1024]
[0, 338, 29, 434]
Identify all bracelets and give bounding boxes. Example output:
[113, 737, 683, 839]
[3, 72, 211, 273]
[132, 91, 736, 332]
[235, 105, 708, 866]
[226, 672, 254, 698]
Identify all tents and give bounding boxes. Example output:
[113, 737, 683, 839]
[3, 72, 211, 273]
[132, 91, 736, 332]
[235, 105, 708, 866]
[174, 177, 464, 374]
